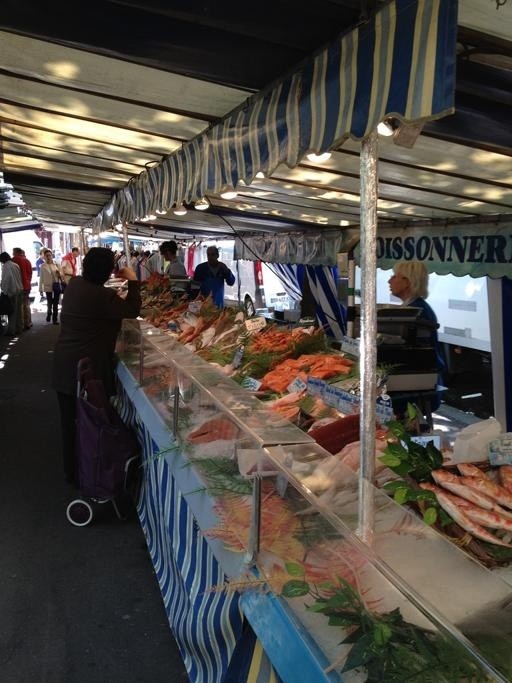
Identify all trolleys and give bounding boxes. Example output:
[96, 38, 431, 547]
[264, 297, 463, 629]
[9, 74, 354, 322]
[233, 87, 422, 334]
[67, 358, 140, 527]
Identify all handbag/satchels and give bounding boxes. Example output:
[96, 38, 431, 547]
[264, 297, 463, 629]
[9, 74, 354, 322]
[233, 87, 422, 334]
[53, 276, 67, 292]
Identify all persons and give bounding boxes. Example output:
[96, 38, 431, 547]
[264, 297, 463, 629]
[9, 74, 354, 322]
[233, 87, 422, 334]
[55, 247, 142, 508]
[389, 260, 446, 411]
[0, 251, 23, 339]
[11, 248, 33, 331]
[191, 246, 236, 308]
[36, 248, 49, 276]
[112, 249, 163, 280]
[39, 249, 65, 325]
[61, 247, 80, 292]
[159, 241, 187, 276]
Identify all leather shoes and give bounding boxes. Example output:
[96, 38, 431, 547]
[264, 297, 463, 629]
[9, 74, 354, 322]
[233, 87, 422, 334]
[46, 313, 52, 321]
[53, 320, 59, 324]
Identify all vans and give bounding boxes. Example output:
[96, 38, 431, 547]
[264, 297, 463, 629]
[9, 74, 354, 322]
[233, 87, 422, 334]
[183, 241, 298, 320]
